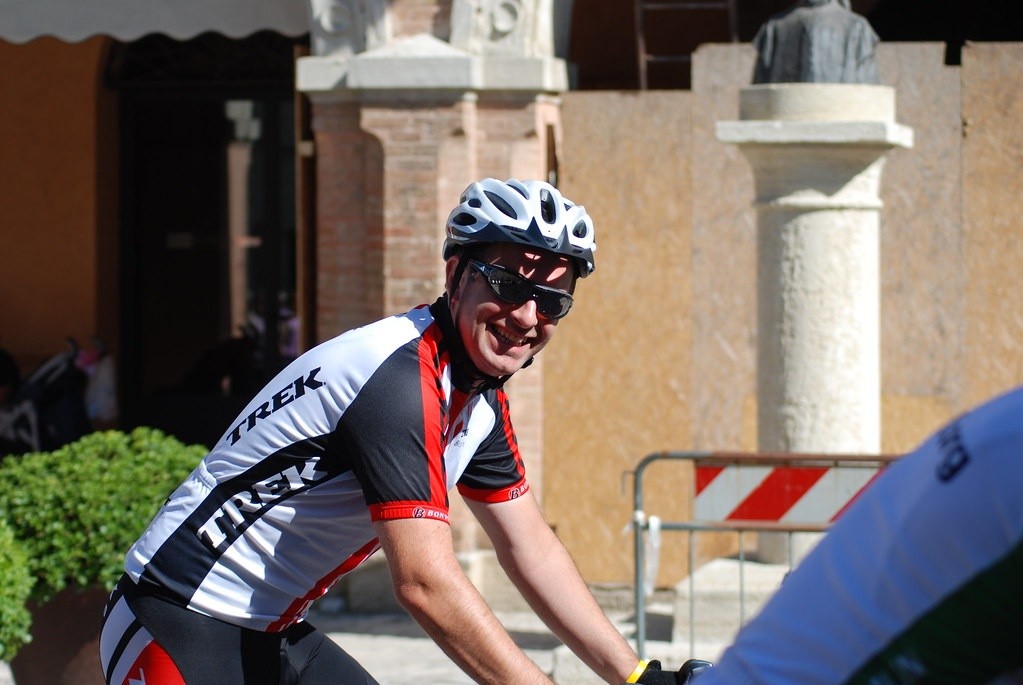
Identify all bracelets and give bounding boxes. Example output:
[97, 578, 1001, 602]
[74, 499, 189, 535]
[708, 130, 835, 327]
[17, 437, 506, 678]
[626, 661, 647, 683]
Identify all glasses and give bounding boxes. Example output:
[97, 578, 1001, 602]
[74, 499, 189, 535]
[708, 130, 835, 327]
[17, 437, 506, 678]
[467, 258, 574, 320]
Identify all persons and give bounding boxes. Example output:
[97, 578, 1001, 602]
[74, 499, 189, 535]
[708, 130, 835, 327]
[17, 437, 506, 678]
[222, 283, 297, 407]
[696, 385, 1023, 685]
[98, 178, 712, 685]
[0, 348, 57, 458]
[83, 336, 114, 427]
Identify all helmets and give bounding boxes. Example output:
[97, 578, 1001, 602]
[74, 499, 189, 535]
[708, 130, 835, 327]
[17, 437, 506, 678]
[442, 177, 597, 278]
